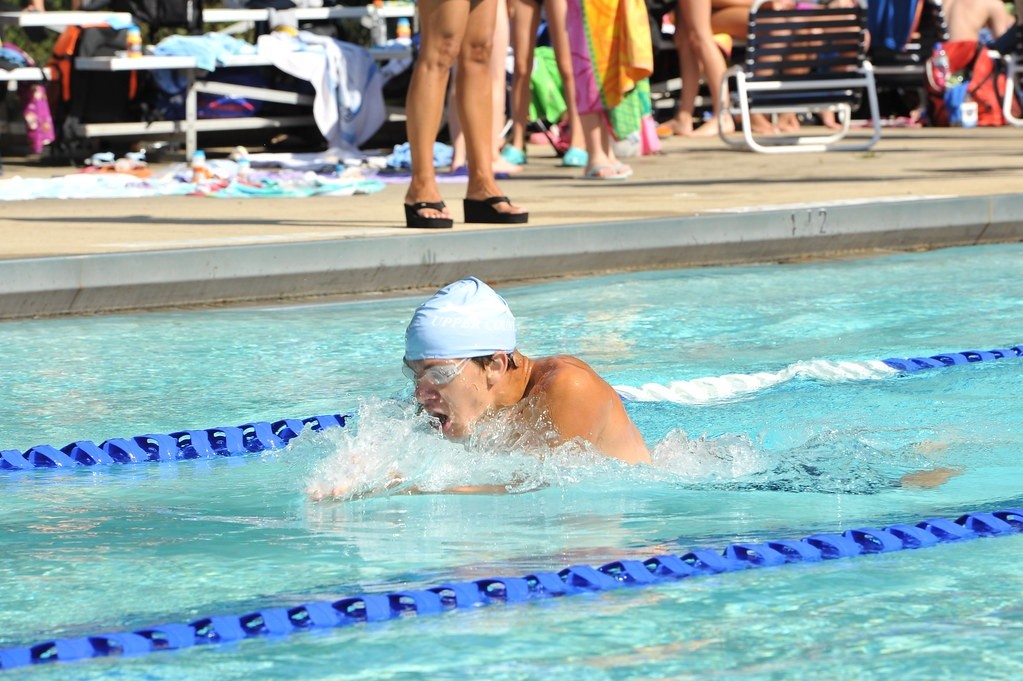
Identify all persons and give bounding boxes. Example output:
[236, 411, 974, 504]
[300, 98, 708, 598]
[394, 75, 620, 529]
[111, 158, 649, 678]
[564, 0, 634, 179]
[403, 274, 652, 466]
[506, 0, 589, 166]
[403, 0, 530, 229]
[660, 0, 736, 139]
[445, 0, 525, 175]
[711, 0, 871, 137]
[940, 0, 1012, 73]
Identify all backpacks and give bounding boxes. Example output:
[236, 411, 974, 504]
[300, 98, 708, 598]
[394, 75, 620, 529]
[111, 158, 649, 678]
[46, 23, 146, 125]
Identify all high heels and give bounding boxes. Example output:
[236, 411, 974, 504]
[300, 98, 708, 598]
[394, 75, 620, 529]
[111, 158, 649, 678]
[463, 196, 529, 223]
[404, 202, 454, 228]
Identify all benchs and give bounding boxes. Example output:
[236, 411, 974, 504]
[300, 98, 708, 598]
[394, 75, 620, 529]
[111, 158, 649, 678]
[0, 0, 738, 161]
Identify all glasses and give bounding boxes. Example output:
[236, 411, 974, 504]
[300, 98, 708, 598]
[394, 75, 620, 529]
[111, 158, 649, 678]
[401, 357, 471, 386]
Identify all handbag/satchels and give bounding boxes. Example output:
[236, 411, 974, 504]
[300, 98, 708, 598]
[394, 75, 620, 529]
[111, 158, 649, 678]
[924, 35, 1021, 128]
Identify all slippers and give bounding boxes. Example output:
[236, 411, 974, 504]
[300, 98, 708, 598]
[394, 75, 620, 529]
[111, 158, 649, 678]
[574, 164, 633, 180]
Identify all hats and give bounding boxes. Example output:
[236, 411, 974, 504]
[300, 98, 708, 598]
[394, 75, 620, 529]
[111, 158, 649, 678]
[407, 277, 518, 361]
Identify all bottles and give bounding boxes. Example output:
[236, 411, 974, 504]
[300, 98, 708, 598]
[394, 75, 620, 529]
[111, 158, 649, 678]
[126, 25, 142, 58]
[238, 158, 251, 184]
[397, 18, 411, 39]
[192, 150, 207, 182]
[933, 43, 950, 85]
[979, 29, 993, 46]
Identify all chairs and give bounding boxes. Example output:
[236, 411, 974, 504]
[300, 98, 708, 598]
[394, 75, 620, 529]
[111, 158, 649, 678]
[717, 0, 1023, 154]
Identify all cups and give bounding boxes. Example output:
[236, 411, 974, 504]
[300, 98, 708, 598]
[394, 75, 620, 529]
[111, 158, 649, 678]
[958, 101, 979, 128]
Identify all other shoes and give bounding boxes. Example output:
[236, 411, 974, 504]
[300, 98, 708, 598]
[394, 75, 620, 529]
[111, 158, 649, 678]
[500, 143, 526, 165]
[564, 146, 590, 167]
